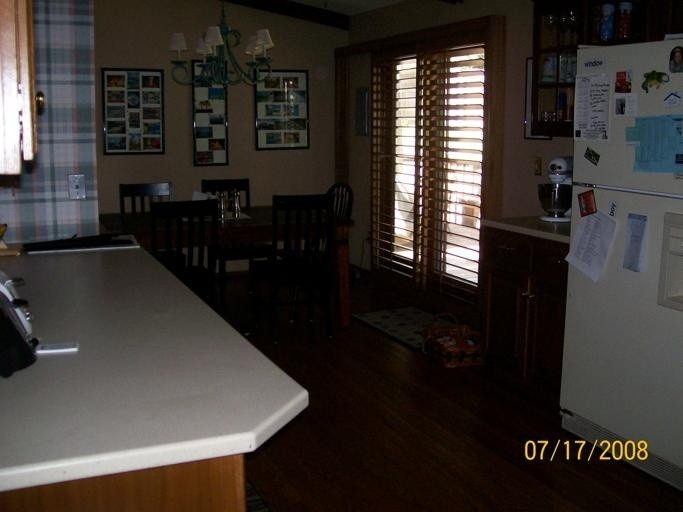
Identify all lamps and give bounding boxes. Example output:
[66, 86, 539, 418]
[168, 0, 276, 86]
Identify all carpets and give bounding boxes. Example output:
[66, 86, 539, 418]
[352, 305, 453, 349]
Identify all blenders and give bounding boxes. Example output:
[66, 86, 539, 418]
[537, 156, 572, 223]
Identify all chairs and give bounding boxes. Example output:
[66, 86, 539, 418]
[119, 177, 354, 319]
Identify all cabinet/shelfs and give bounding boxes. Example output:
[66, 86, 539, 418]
[0, 1, 44, 176]
[0, 453, 246, 511]
[480, 226, 570, 426]
[530, 0, 682, 138]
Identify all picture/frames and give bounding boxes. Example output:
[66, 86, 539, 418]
[191, 58, 229, 166]
[101, 67, 165, 156]
[253, 68, 309, 151]
[524, 57, 553, 140]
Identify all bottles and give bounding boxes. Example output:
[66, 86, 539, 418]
[597, 2, 633, 44]
[543, 7, 576, 49]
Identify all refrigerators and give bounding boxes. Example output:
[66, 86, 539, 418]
[557, 39, 682, 493]
